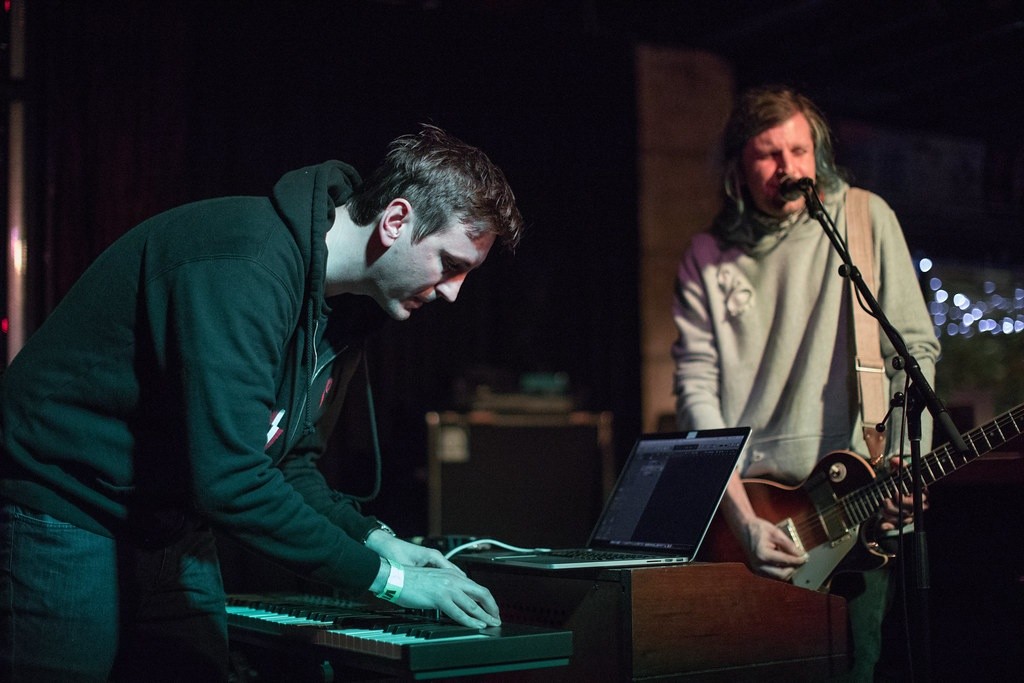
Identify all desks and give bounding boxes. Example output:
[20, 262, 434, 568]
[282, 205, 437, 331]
[426, 409, 615, 537]
[453, 554, 853, 683]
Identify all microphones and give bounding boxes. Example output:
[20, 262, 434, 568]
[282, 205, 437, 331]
[778, 174, 812, 202]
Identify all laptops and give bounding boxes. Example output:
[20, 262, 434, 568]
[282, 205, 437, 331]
[455, 425, 753, 570]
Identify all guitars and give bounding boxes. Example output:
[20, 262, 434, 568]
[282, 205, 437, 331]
[695, 402, 1024, 595]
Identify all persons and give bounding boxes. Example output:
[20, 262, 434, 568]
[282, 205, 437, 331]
[0, 121, 524, 683]
[672, 87, 942, 683]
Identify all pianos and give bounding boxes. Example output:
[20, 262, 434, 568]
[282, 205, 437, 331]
[223, 589, 575, 683]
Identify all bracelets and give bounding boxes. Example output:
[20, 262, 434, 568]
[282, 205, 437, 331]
[376, 555, 405, 603]
[363, 519, 396, 544]
